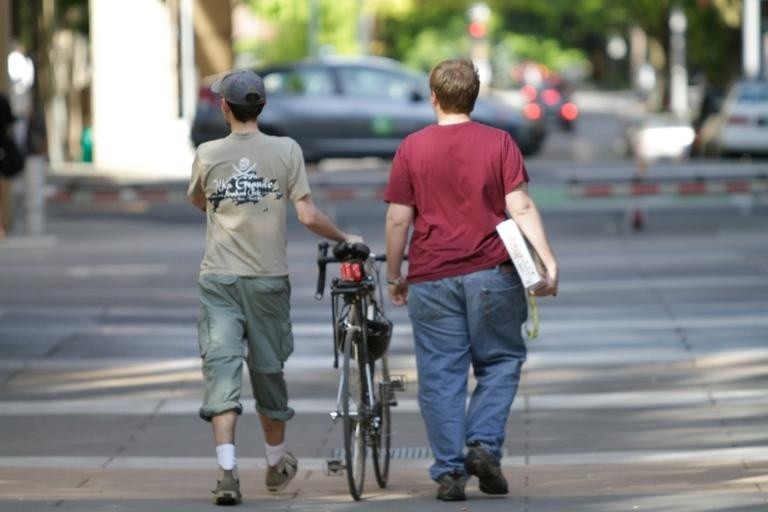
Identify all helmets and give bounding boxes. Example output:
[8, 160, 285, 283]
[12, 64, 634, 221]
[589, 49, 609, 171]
[335, 298, 393, 363]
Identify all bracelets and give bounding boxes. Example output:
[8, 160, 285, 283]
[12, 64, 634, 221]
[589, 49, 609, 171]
[387, 276, 403, 287]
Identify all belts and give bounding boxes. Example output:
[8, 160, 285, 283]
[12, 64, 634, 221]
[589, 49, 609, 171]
[493, 259, 513, 269]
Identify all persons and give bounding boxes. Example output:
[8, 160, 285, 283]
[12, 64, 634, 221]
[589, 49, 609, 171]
[385, 58, 559, 502]
[186, 68, 364, 505]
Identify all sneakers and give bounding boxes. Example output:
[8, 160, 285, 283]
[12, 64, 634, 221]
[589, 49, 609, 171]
[265, 451, 297, 492]
[436, 446, 509, 501]
[211, 464, 242, 506]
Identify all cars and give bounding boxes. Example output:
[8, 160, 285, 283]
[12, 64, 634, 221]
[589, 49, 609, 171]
[714, 80, 768, 163]
[187, 57, 546, 167]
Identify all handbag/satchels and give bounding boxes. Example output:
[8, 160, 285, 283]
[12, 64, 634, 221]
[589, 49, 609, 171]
[496, 219, 548, 291]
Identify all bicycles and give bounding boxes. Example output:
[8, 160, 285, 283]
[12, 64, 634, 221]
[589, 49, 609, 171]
[310, 240, 412, 498]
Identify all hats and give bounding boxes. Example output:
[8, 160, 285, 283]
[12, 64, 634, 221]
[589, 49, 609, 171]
[211, 69, 266, 105]
